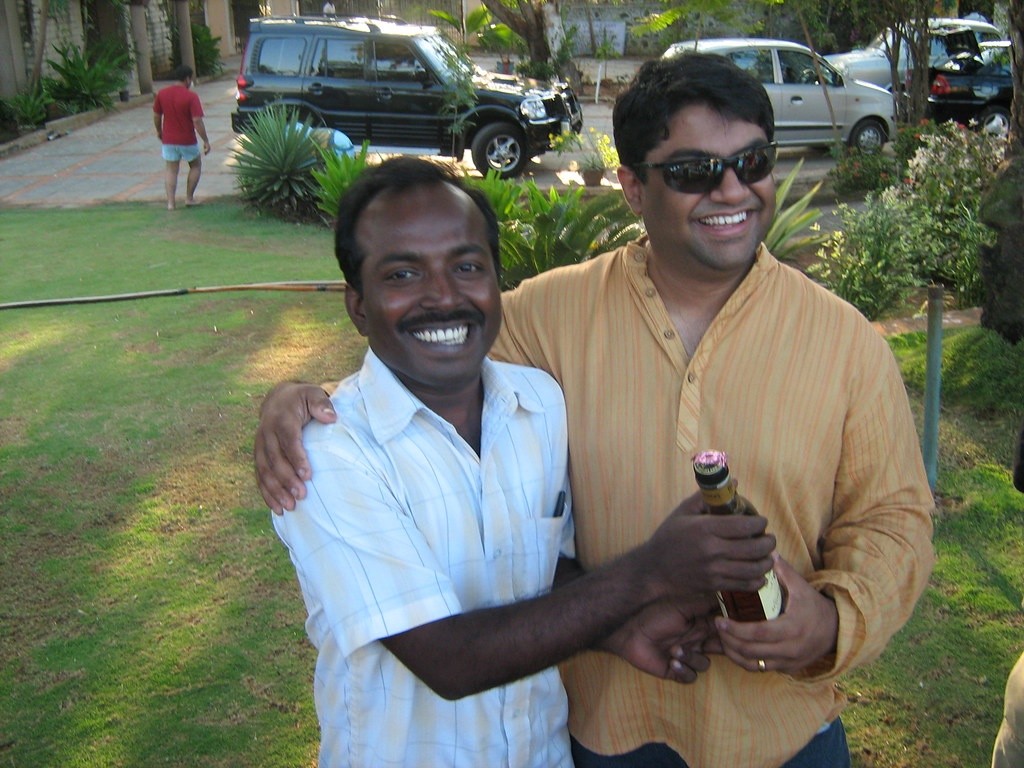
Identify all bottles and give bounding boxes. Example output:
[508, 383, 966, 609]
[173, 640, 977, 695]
[691, 449, 783, 624]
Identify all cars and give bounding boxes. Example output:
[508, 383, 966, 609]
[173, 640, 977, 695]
[819, 18, 1004, 100]
[657, 37, 903, 155]
[905, 39, 1015, 145]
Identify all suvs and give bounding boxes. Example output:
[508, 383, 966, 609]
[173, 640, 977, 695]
[230, 12, 584, 181]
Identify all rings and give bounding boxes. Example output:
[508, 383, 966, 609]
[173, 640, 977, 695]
[758, 659, 765, 670]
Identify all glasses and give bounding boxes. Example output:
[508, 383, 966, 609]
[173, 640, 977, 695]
[628, 141, 779, 194]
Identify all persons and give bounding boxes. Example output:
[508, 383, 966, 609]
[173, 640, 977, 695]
[255, 52, 937, 768]
[153, 64, 211, 210]
[323, 0, 338, 18]
